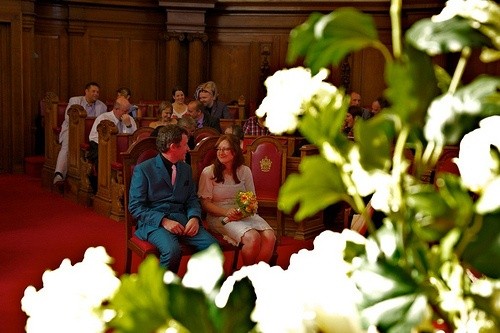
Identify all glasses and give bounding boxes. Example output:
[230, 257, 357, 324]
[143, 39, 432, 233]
[216, 148, 233, 152]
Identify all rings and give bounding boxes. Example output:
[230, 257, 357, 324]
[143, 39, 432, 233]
[229, 213, 231, 215]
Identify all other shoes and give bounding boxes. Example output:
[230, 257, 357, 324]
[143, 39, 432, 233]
[54, 174, 63, 184]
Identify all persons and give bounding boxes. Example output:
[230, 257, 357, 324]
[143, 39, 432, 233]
[194, 81, 232, 129]
[88, 97, 137, 191]
[128, 125, 219, 274]
[242, 96, 274, 136]
[53, 82, 107, 195]
[178, 101, 222, 139]
[117, 87, 142, 118]
[345, 91, 390, 140]
[149, 100, 178, 129]
[171, 87, 188, 120]
[196, 134, 275, 266]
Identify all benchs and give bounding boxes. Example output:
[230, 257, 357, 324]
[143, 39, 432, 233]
[41, 90, 459, 241]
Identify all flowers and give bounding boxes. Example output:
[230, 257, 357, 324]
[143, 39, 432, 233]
[221, 192, 258, 224]
[21, 0, 500, 333]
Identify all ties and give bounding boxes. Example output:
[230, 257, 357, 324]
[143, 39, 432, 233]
[171, 164, 177, 185]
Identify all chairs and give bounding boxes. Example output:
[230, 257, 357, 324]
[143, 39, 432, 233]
[119, 136, 198, 274]
[189, 134, 279, 274]
[246, 134, 288, 236]
